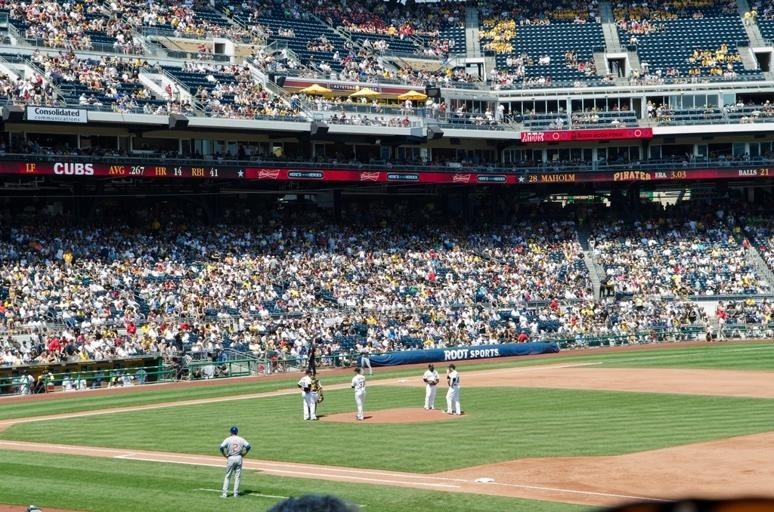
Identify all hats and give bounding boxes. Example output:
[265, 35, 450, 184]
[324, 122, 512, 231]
[230, 427, 238, 432]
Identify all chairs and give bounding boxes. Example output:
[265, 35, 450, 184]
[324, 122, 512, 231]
[240, 4, 610, 84]
[615, 23, 624, 47]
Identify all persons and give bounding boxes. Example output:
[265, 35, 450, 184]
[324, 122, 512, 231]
[421, 363, 440, 410]
[218, 426, 252, 499]
[443, 363, 461, 416]
[310, 376, 322, 416]
[1, 195, 773, 394]
[297, 370, 319, 421]
[2, 2, 773, 164]
[350, 367, 366, 420]
